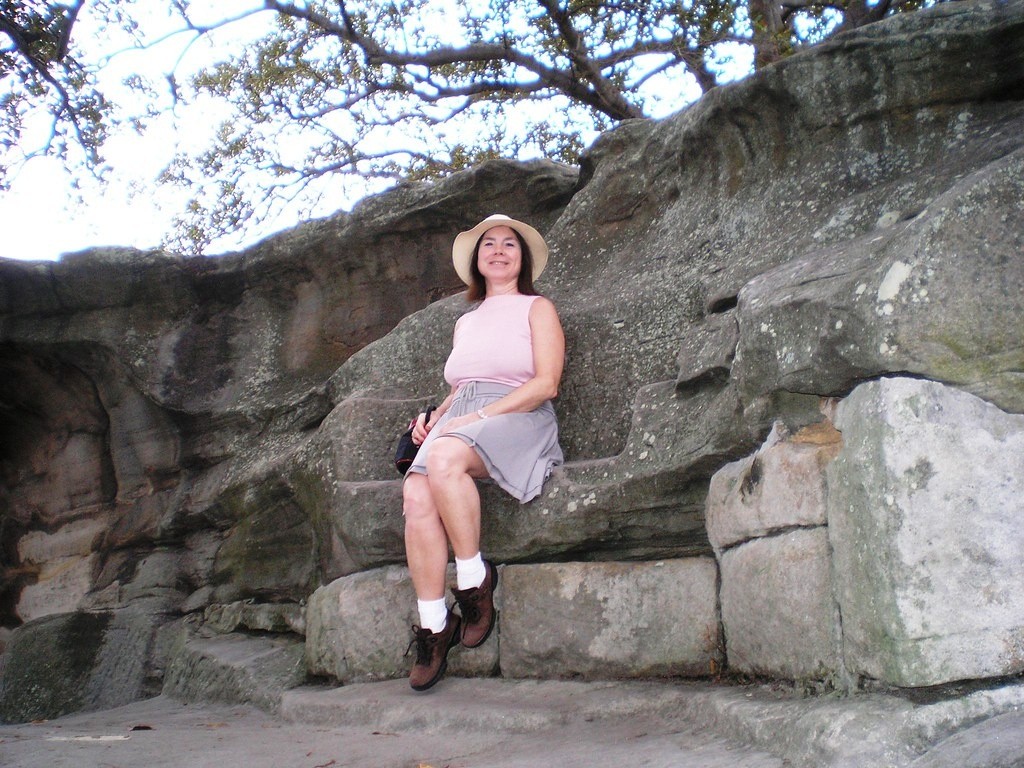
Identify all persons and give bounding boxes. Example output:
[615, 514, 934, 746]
[403, 214, 566, 693]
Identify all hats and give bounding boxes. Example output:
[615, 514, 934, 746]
[452, 214, 549, 287]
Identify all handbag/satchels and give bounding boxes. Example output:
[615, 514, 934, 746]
[396, 405, 437, 475]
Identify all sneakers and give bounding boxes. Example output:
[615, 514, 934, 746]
[403, 605, 462, 691]
[451, 557, 499, 648]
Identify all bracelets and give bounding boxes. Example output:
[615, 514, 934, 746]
[477, 410, 487, 419]
